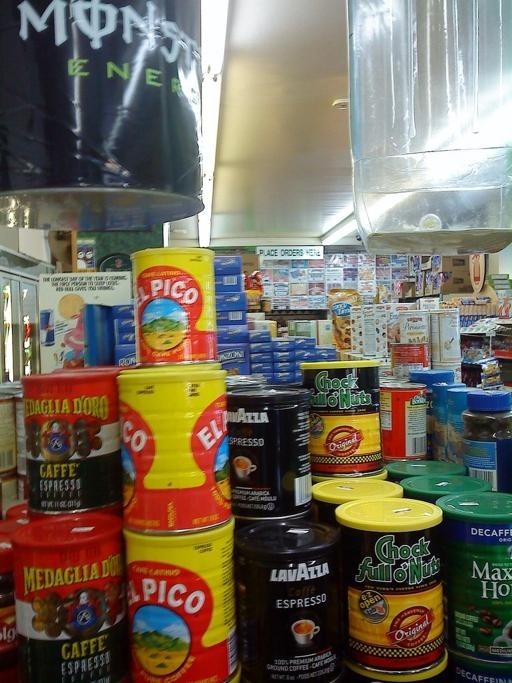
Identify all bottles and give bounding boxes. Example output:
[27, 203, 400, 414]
[10, 247, 511, 683]
